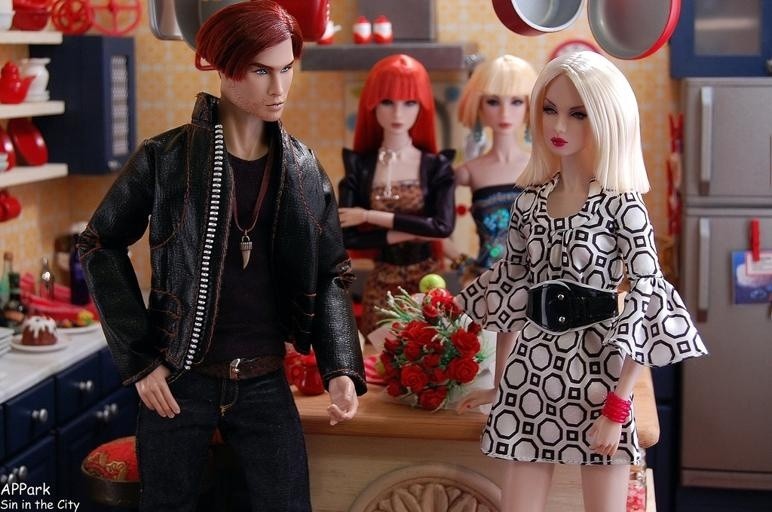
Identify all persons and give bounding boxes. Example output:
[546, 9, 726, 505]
[74, 0, 368, 512]
[333, 54, 458, 344]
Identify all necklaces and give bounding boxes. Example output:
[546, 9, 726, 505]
[228, 143, 272, 269]
[377, 140, 411, 201]
